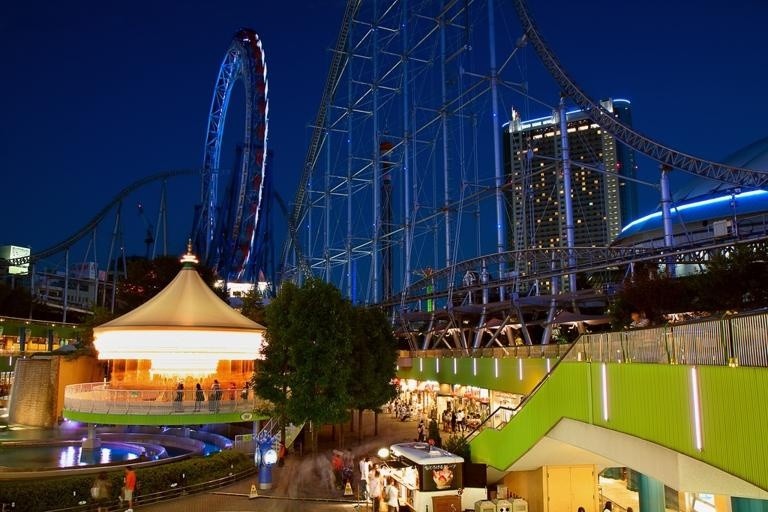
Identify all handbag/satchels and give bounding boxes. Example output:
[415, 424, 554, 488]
[90, 487, 100, 497]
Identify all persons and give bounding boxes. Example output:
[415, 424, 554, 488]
[602, 501, 614, 511]
[119, 465, 137, 511]
[93, 471, 113, 511]
[276, 441, 285, 468]
[173, 384, 185, 411]
[211, 379, 223, 413]
[514, 330, 523, 345]
[229, 382, 237, 413]
[241, 382, 250, 409]
[390, 397, 484, 450]
[629, 311, 650, 329]
[331, 448, 400, 512]
[193, 382, 205, 411]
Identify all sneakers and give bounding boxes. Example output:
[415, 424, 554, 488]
[119, 496, 134, 512]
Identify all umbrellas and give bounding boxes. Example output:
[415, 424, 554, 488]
[475, 319, 538, 331]
[421, 321, 478, 337]
[391, 326, 422, 336]
[546, 308, 610, 326]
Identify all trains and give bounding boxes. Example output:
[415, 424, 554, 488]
[607, 189, 768, 252]
